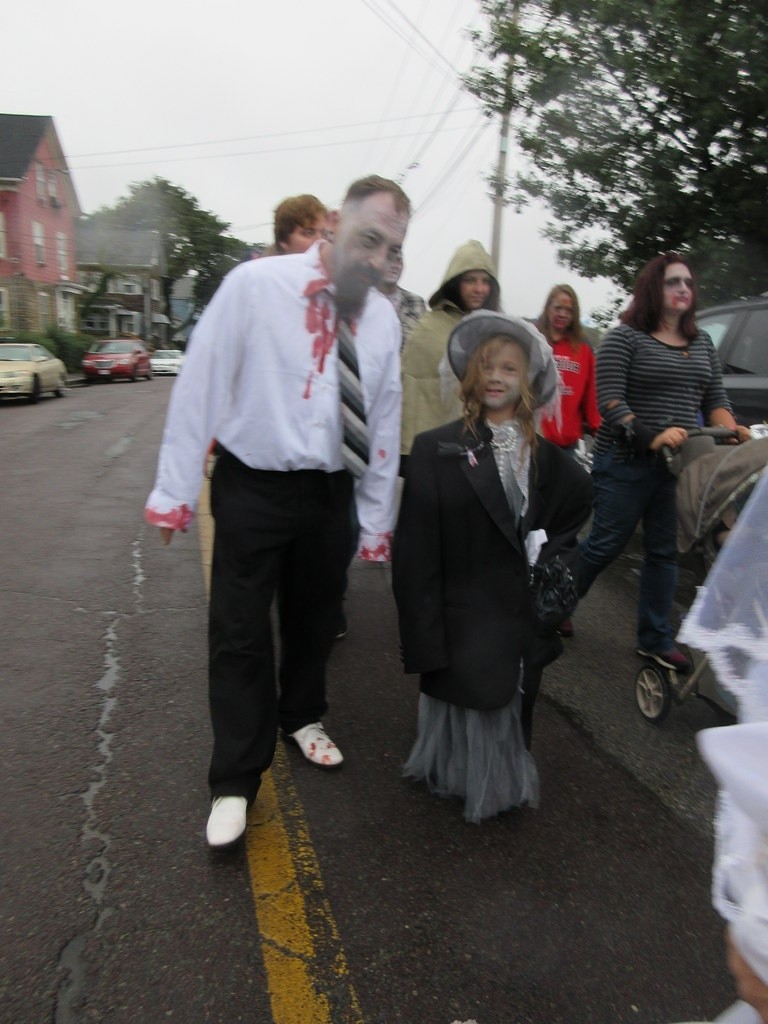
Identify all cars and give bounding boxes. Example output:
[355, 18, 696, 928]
[0, 343, 66, 403]
[81, 340, 154, 382]
[150, 350, 184, 373]
[692, 292, 768, 428]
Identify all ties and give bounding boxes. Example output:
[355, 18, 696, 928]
[326, 288, 368, 479]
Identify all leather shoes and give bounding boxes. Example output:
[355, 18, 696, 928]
[289, 721, 345, 769]
[206, 794, 248, 847]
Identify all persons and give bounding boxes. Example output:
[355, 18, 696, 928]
[260, 194, 350, 645]
[559, 251, 753, 670]
[390, 308, 593, 825]
[375, 249, 425, 352]
[401, 240, 503, 458]
[144, 175, 414, 847]
[533, 284, 600, 552]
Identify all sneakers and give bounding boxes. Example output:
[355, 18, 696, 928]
[556, 618, 574, 636]
[637, 642, 691, 671]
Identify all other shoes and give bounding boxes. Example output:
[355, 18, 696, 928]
[336, 613, 348, 638]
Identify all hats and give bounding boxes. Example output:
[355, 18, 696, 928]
[447, 310, 557, 410]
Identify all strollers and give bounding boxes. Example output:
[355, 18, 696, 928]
[633, 429, 768, 723]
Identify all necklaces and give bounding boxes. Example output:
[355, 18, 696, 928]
[491, 427, 517, 478]
